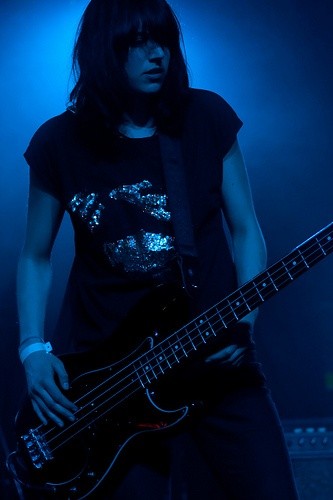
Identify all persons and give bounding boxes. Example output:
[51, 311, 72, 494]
[14, 0, 299, 500]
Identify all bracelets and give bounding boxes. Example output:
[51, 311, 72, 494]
[18, 337, 52, 362]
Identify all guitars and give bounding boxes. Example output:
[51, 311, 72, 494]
[11, 221, 333, 500]
